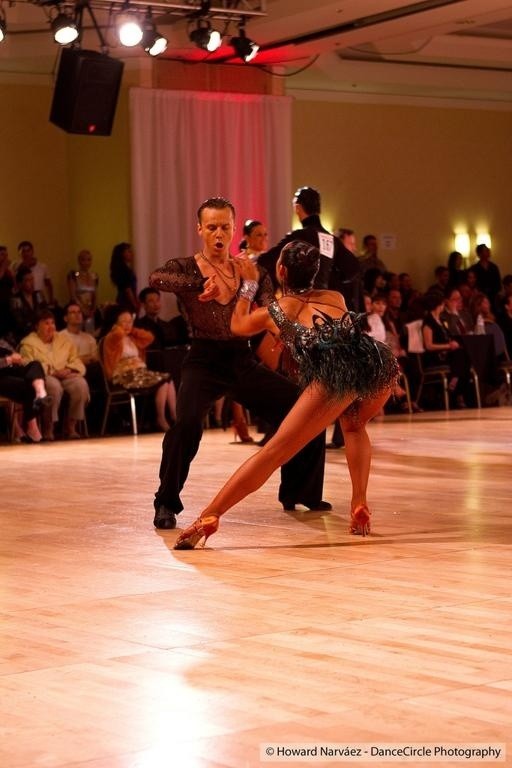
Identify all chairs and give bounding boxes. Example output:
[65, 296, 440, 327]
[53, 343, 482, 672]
[0, 395, 23, 442]
[483, 320, 512, 406]
[406, 319, 452, 410]
[97, 335, 146, 435]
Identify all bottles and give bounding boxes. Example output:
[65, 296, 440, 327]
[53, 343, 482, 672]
[476, 314, 484, 335]
[85, 318, 95, 337]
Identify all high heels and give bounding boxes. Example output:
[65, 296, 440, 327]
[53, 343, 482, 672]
[174, 511, 217, 549]
[348, 506, 371, 536]
[232, 421, 253, 443]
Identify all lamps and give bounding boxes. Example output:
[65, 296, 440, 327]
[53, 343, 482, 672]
[142, 24, 168, 57]
[230, 27, 260, 63]
[0, 13, 7, 42]
[190, 24, 222, 52]
[116, 13, 144, 48]
[50, 12, 78, 45]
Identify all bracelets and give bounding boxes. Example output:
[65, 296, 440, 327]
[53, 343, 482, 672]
[241, 280, 259, 303]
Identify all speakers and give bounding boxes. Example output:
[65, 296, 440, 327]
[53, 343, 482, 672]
[47, 45, 126, 138]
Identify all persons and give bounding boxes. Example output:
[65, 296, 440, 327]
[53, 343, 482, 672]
[174, 241, 400, 549]
[0, 233, 223, 442]
[149, 198, 332, 528]
[334, 229, 512, 418]
[225, 220, 270, 444]
[253, 187, 360, 448]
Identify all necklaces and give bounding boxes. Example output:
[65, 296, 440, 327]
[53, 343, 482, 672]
[198, 251, 238, 294]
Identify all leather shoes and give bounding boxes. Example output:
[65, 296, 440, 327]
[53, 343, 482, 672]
[277, 492, 332, 514]
[326, 441, 344, 450]
[153, 498, 179, 529]
[32, 396, 52, 413]
